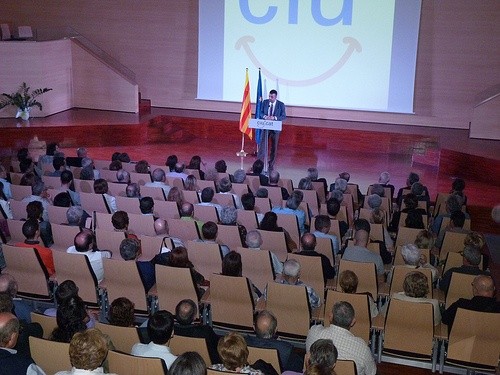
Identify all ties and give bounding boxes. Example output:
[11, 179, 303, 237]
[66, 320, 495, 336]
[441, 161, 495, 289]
[269, 103, 273, 116]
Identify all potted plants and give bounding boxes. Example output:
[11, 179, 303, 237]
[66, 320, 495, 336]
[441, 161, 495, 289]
[0, 81, 52, 121]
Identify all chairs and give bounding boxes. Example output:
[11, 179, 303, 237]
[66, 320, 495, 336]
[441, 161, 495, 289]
[0, 155, 500, 375]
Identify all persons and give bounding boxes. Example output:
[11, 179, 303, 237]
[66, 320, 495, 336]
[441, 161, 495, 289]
[44, 280, 96, 328]
[305, 301, 377, 375]
[260, 89, 286, 170]
[0, 273, 43, 359]
[393, 270, 441, 326]
[167, 351, 207, 375]
[244, 309, 304, 372]
[55, 329, 116, 375]
[131, 311, 178, 369]
[281, 339, 338, 375]
[339, 270, 380, 317]
[174, 298, 222, 364]
[108, 297, 151, 344]
[51, 297, 90, 343]
[209, 332, 279, 375]
[0, 312, 46, 375]
[0, 144, 492, 319]
[442, 276, 500, 330]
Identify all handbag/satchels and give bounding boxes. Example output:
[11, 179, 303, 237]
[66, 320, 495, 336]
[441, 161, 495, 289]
[149, 237, 176, 264]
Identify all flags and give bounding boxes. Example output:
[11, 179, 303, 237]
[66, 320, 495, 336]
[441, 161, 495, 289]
[255, 70, 263, 144]
[239, 70, 252, 139]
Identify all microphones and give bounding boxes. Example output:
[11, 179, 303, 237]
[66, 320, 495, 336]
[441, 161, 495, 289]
[267, 105, 270, 108]
